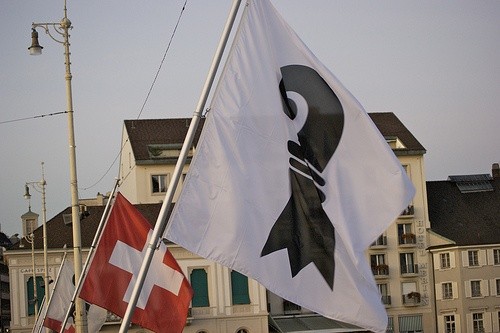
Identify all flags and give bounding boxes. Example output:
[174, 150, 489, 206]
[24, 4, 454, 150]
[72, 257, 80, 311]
[43, 257, 107, 333]
[33, 300, 47, 333]
[78, 191, 195, 333]
[163, 0, 416, 333]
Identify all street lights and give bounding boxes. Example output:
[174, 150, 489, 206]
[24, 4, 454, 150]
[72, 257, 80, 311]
[18, 220, 40, 326]
[28, 1, 90, 333]
[24, 161, 51, 305]
